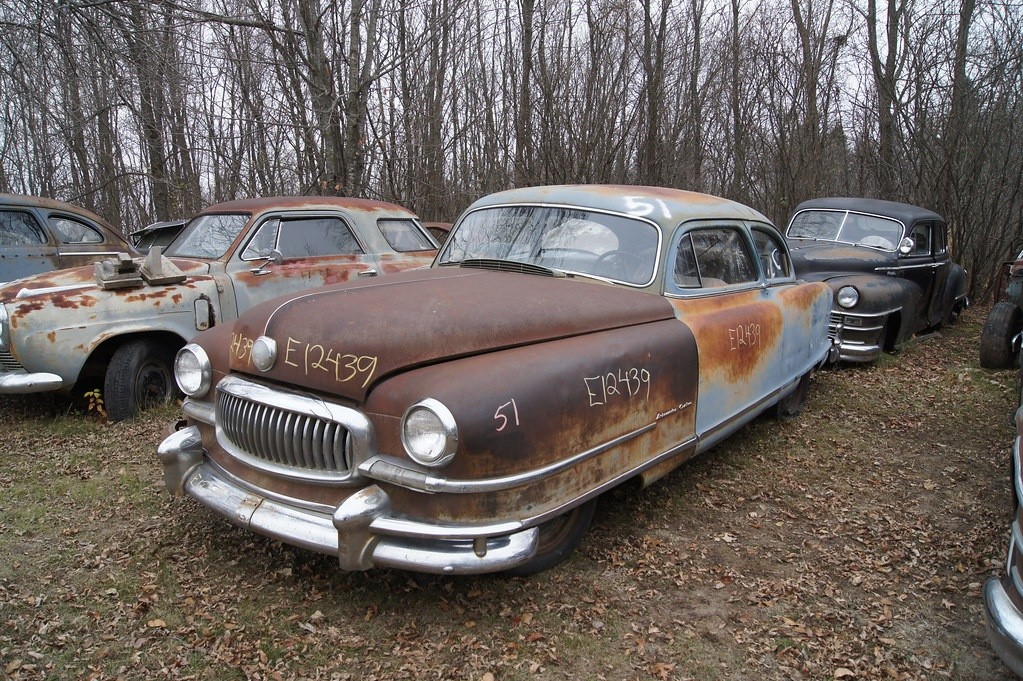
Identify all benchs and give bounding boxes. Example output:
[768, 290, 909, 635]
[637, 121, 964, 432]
[541, 257, 727, 287]
[860, 229, 927, 252]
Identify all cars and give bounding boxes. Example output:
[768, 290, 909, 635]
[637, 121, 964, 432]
[1, 193, 459, 425]
[0, 189, 148, 287]
[761, 194, 970, 367]
[157, 183, 842, 586]
